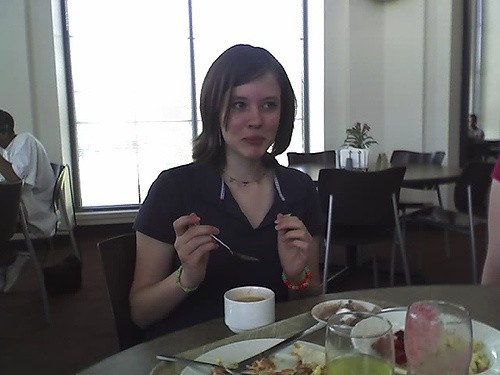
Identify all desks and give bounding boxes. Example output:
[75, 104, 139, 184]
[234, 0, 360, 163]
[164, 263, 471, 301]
[283, 160, 480, 283]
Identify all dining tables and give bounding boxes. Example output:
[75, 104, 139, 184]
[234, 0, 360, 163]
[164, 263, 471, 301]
[79, 284, 500, 375]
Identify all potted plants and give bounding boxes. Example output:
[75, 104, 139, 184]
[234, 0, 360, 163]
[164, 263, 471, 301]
[336, 122, 379, 171]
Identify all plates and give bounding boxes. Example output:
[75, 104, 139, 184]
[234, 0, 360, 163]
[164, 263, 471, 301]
[181, 338, 325, 375]
[350, 308, 500, 375]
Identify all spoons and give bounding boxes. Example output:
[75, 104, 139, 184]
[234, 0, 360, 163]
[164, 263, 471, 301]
[194, 222, 260, 262]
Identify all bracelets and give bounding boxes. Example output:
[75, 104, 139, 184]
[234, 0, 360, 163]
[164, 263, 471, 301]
[281, 265, 311, 290]
[176, 265, 199, 294]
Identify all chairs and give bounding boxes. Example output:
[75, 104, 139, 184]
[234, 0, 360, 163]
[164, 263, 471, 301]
[316, 166, 412, 295]
[391, 150, 454, 254]
[287, 150, 336, 167]
[95, 233, 168, 351]
[0, 162, 80, 319]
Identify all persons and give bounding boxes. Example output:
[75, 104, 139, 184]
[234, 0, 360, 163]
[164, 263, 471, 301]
[0, 110, 57, 292]
[469, 114, 485, 139]
[127, 45, 324, 343]
[480, 157, 500, 285]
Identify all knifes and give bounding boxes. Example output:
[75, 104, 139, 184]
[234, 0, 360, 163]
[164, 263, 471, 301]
[236, 322, 325, 366]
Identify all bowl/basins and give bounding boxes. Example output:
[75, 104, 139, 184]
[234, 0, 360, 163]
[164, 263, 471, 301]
[310, 299, 384, 337]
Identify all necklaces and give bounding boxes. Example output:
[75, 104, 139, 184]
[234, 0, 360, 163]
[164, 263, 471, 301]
[225, 166, 269, 184]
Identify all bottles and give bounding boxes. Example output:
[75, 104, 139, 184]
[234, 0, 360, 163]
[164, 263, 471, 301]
[346, 151, 353, 171]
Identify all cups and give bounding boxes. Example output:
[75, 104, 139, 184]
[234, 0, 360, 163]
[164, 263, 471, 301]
[324, 312, 395, 375]
[224, 286, 276, 334]
[404, 300, 473, 374]
